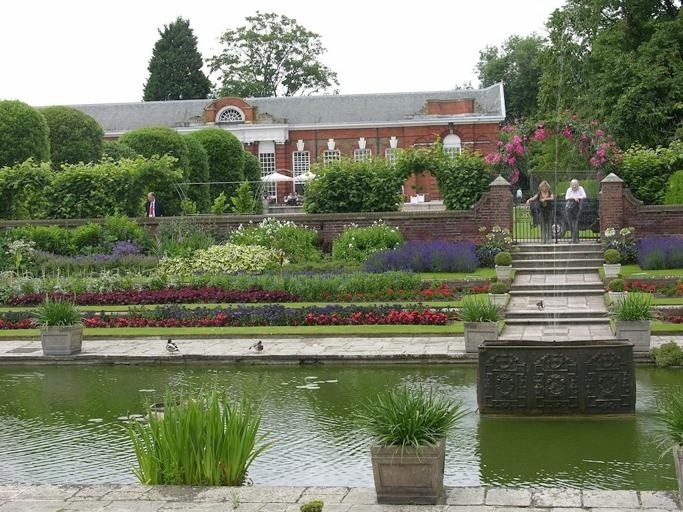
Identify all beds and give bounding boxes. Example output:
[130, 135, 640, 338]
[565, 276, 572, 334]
[262, 171, 320, 204]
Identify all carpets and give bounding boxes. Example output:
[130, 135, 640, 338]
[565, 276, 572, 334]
[150, 203, 153, 214]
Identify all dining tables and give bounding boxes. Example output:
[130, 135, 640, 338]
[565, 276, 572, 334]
[456, 293, 502, 353]
[607, 278, 628, 311]
[30, 295, 83, 357]
[494, 251, 512, 280]
[488, 282, 509, 307]
[603, 249, 621, 278]
[609, 288, 652, 352]
[352, 383, 472, 505]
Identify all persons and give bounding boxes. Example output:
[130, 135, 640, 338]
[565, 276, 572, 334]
[146, 192, 162, 217]
[565, 179, 586, 244]
[266, 192, 298, 206]
[526, 181, 554, 244]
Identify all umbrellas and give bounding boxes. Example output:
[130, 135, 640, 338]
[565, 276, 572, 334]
[569, 238, 580, 244]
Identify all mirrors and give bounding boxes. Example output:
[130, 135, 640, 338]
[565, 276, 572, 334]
[536, 299, 544, 310]
[165, 338, 180, 354]
[248, 340, 264, 354]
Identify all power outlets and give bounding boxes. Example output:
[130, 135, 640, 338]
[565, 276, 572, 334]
[530, 198, 599, 238]
